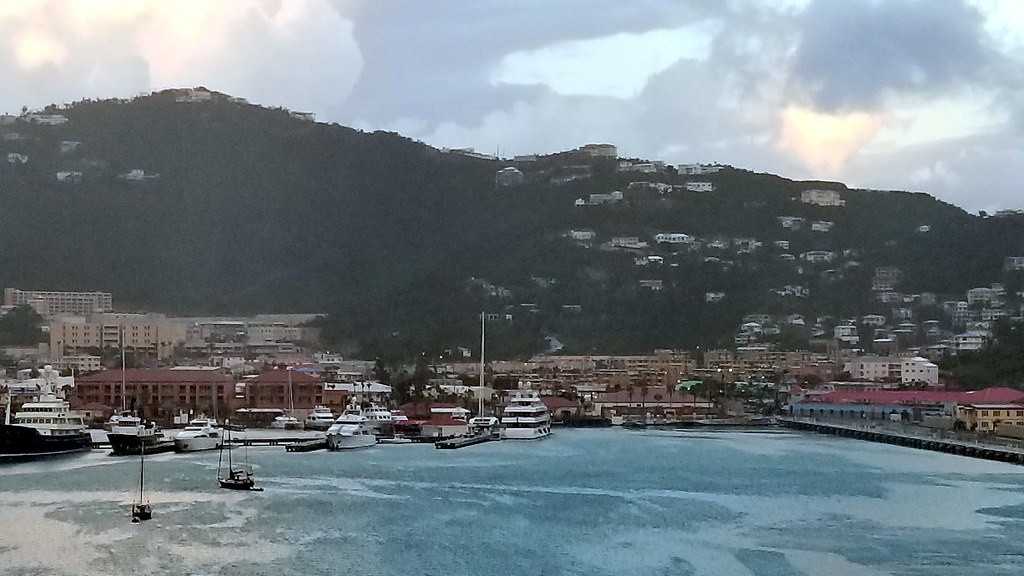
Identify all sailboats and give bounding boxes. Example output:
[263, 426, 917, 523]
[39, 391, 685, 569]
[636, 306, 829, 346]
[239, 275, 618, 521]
[215, 394, 256, 490]
[133, 433, 152, 523]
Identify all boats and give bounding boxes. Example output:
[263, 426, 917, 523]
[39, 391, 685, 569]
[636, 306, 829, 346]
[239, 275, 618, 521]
[380, 409, 423, 437]
[271, 408, 304, 430]
[361, 403, 392, 428]
[499, 381, 553, 440]
[324, 396, 378, 449]
[107, 408, 175, 457]
[1, 378, 93, 460]
[173, 412, 224, 453]
[305, 404, 335, 432]
[379, 433, 412, 444]
[437, 423, 500, 448]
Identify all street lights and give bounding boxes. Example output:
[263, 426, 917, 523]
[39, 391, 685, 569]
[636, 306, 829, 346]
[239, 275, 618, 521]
[717, 368, 733, 419]
